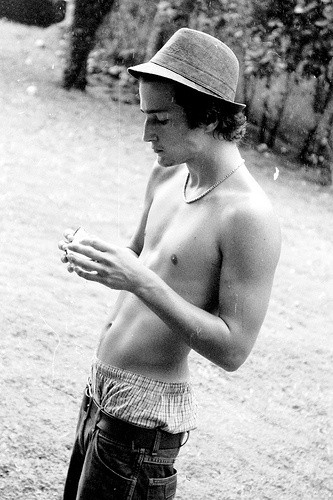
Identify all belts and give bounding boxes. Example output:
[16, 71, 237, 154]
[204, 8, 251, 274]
[83, 387, 184, 450]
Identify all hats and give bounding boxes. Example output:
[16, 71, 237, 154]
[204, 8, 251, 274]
[128, 28, 246, 115]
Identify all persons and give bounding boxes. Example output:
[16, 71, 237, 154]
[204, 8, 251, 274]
[61, 0, 120, 89]
[55, 28, 283, 500]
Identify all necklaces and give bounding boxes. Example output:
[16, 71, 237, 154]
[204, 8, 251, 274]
[182, 156, 245, 204]
